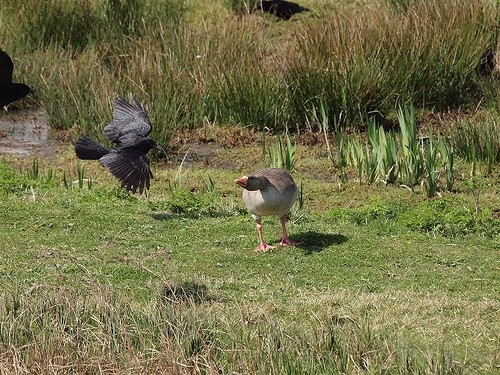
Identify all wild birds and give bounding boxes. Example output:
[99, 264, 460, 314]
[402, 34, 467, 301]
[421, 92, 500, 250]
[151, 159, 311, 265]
[73, 90, 162, 196]
[234, 167, 301, 253]
[0, 49, 35, 112]
